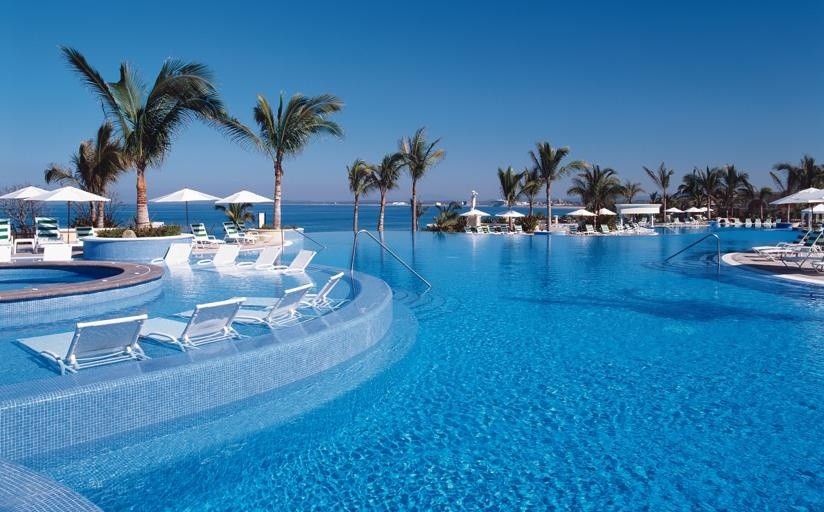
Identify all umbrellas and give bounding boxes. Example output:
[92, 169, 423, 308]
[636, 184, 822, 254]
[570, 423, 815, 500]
[666, 206, 714, 214]
[215, 190, 275, 230]
[23, 186, 111, 244]
[769, 185, 824, 232]
[593, 208, 617, 224]
[0, 186, 50, 234]
[801, 204, 824, 214]
[148, 185, 224, 233]
[494, 210, 525, 230]
[567, 209, 598, 232]
[460, 209, 490, 226]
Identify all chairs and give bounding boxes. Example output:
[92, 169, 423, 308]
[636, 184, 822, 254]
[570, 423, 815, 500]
[578, 222, 654, 236]
[189, 220, 259, 249]
[750, 243, 824, 276]
[138, 297, 245, 353]
[227, 270, 345, 315]
[150, 242, 317, 276]
[0, 217, 94, 262]
[172, 283, 314, 331]
[16, 313, 151, 378]
